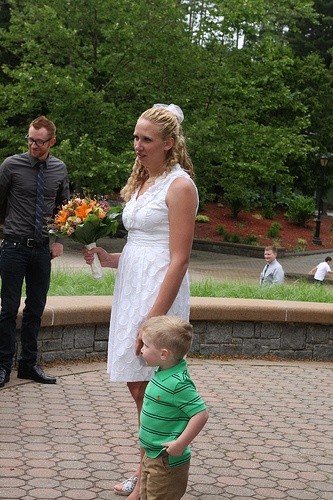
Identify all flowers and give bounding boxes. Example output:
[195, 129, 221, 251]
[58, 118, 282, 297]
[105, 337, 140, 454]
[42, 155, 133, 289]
[43, 187, 124, 279]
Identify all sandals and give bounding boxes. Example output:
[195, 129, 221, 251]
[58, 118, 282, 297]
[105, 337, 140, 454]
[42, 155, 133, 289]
[114, 475, 138, 496]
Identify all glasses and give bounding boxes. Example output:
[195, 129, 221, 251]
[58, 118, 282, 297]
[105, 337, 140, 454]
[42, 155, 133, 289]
[25, 137, 55, 147]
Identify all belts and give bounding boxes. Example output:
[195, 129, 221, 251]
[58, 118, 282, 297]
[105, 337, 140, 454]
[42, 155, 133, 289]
[3, 236, 50, 249]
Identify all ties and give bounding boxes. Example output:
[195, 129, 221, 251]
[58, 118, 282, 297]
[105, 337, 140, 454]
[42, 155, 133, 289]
[35, 164, 45, 251]
[261, 265, 269, 286]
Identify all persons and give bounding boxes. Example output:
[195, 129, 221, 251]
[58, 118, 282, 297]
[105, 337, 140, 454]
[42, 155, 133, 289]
[260, 245, 285, 288]
[0, 115, 70, 387]
[308, 256, 333, 286]
[139, 315, 209, 500]
[82, 103, 200, 500]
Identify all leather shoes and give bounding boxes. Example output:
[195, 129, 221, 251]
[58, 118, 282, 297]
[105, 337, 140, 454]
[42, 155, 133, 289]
[18, 365, 57, 384]
[0, 367, 11, 388]
